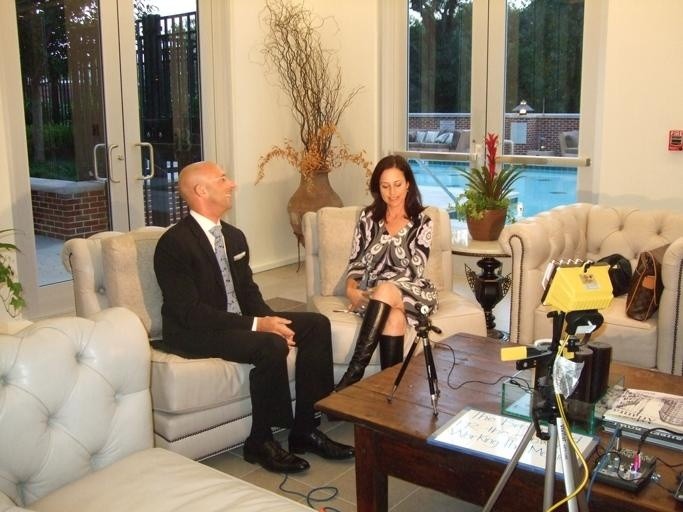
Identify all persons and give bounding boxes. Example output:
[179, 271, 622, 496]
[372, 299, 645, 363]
[327, 154, 442, 397]
[149, 159, 356, 474]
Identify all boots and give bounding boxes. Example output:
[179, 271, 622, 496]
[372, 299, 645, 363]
[334, 299, 391, 392]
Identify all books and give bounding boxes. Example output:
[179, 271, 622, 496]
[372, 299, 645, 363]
[602, 385, 683, 453]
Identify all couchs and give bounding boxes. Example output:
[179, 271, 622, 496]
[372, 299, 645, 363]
[1, 307, 330, 512]
[409, 129, 470, 152]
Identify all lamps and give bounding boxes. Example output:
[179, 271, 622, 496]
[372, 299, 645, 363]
[512, 96, 534, 115]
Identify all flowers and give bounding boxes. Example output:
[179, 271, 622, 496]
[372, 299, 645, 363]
[449, 133, 520, 221]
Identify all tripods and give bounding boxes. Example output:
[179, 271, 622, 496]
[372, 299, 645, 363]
[481, 389, 590, 512]
[387, 323, 442, 418]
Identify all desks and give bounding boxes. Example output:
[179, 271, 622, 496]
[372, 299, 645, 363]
[312, 333, 683, 512]
[448, 227, 512, 341]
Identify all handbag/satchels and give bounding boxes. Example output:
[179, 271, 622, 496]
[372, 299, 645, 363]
[626, 243, 670, 321]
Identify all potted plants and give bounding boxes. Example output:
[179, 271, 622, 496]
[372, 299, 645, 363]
[252, 1, 372, 248]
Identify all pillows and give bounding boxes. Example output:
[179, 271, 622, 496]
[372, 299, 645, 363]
[317, 203, 362, 298]
[100, 229, 167, 345]
[415, 130, 454, 145]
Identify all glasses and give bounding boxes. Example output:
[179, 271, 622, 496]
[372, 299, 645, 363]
[206, 175, 226, 184]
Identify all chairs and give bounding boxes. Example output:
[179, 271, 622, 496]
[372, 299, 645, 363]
[299, 206, 488, 385]
[62, 223, 299, 462]
[558, 130, 578, 158]
[500, 201, 683, 375]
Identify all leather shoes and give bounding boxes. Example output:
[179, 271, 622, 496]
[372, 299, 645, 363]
[244, 438, 310, 473]
[289, 428, 356, 459]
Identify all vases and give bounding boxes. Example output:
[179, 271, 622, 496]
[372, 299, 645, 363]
[466, 205, 508, 241]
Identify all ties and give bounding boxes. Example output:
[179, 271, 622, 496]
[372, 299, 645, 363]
[209, 226, 243, 316]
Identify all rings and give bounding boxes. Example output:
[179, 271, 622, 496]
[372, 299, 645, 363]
[360, 303, 367, 311]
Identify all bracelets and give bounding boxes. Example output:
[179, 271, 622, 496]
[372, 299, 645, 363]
[364, 287, 375, 301]
